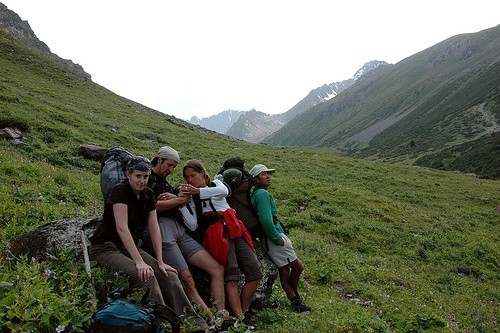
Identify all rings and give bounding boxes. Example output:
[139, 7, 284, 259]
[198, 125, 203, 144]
[186, 186, 188, 188]
[163, 195, 165, 197]
[165, 197, 167, 199]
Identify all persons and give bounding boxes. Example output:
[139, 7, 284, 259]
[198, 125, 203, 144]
[177, 159, 266, 329]
[89, 155, 209, 333]
[140, 145, 239, 330]
[249, 163, 311, 313]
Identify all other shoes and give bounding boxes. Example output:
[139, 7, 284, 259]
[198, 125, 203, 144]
[239, 318, 255, 330]
[293, 303, 311, 311]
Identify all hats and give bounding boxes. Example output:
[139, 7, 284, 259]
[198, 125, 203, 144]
[151, 145, 180, 167]
[249, 164, 276, 177]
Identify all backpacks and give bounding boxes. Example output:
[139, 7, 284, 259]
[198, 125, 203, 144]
[84, 284, 184, 333]
[101, 146, 136, 199]
